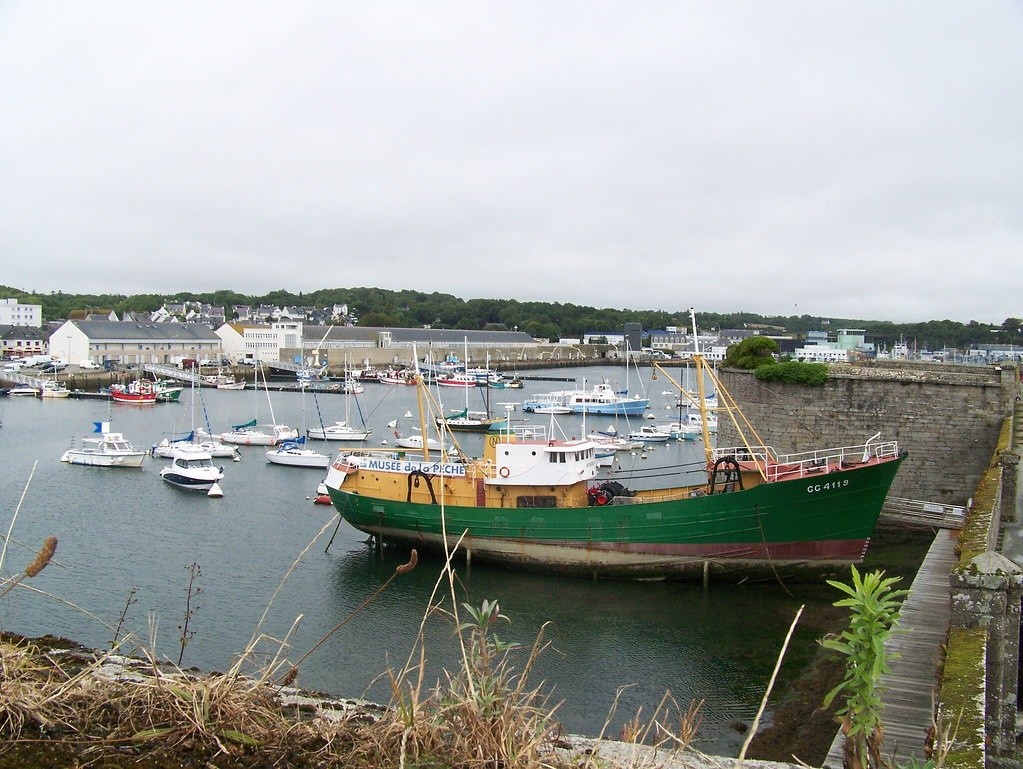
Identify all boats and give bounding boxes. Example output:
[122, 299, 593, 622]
[522, 392, 571, 411]
[685, 414, 718, 432]
[674, 398, 703, 405]
[587, 436, 644, 449]
[531, 408, 574, 416]
[568, 338, 648, 417]
[323, 303, 909, 585]
[0, 351, 526, 509]
[590, 441, 633, 452]
[626, 431, 671, 442]
[592, 449, 617, 467]
[653, 425, 699, 440]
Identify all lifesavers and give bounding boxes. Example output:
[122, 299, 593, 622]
[500, 467, 509, 478]
